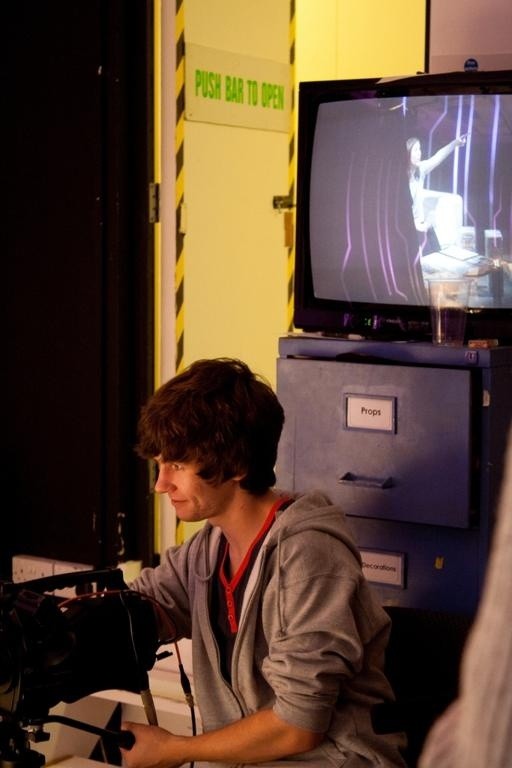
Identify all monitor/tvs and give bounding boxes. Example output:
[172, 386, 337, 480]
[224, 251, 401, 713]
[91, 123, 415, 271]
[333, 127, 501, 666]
[293, 69, 512, 344]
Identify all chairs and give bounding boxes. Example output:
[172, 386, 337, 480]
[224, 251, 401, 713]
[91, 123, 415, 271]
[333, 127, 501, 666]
[0, 522, 158, 725]
[370, 604, 478, 767]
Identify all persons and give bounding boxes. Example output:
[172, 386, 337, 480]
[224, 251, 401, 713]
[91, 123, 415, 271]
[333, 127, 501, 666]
[405, 133, 473, 256]
[115, 356, 409, 768]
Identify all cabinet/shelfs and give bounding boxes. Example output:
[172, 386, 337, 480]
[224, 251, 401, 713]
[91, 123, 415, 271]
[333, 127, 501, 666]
[271, 326, 512, 626]
